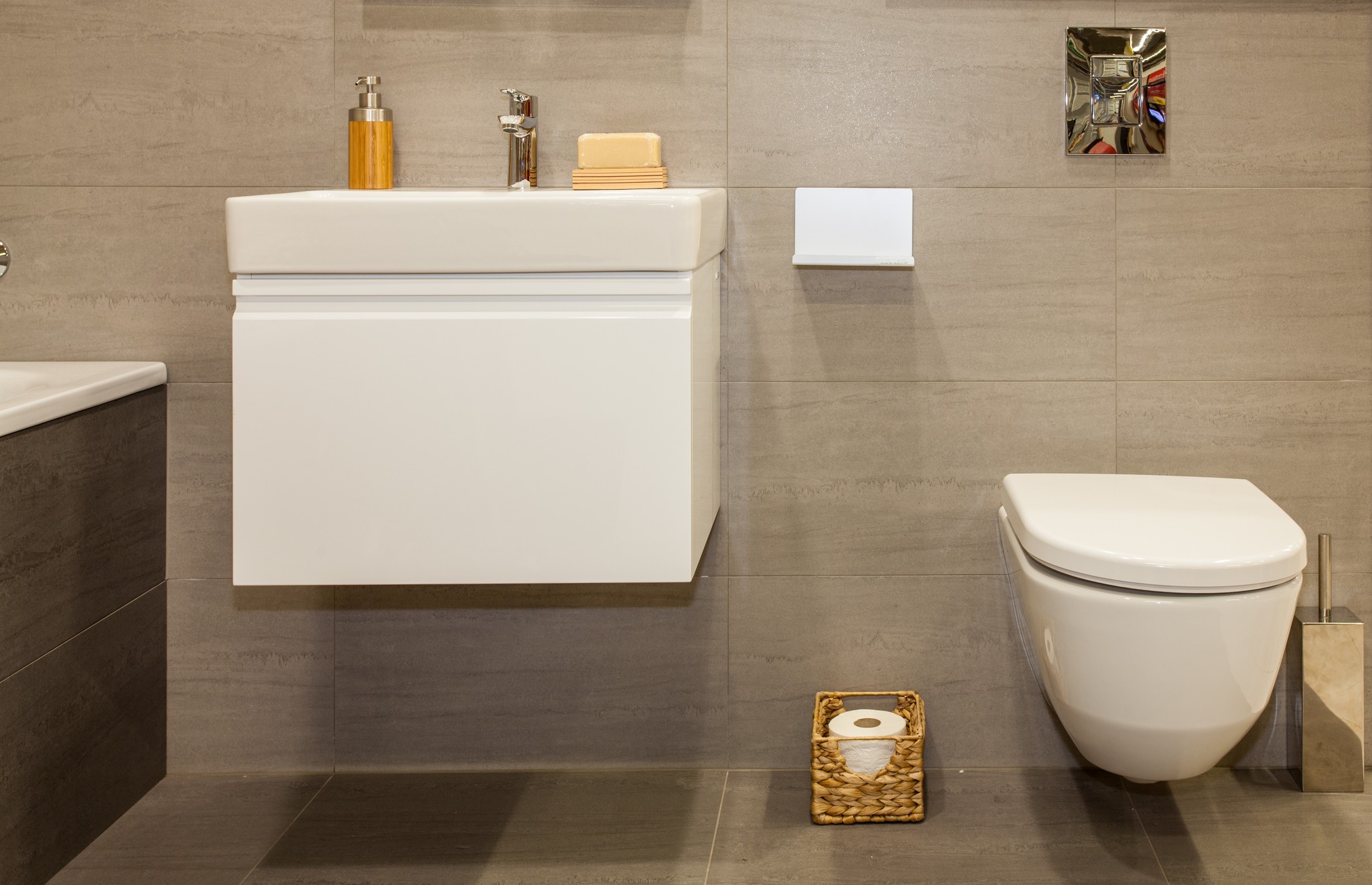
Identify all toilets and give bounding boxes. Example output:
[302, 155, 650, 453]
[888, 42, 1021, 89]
[994, 470, 1309, 786]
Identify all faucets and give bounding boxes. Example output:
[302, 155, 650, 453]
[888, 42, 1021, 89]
[497, 88, 540, 187]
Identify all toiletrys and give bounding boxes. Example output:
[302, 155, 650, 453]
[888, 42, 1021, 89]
[347, 76, 395, 190]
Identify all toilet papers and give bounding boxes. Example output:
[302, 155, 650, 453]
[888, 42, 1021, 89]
[827, 708, 907, 775]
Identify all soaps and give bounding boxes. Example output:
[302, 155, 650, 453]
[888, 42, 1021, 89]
[576, 132, 663, 170]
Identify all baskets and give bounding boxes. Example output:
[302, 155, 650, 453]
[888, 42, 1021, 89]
[809, 689, 927, 824]
